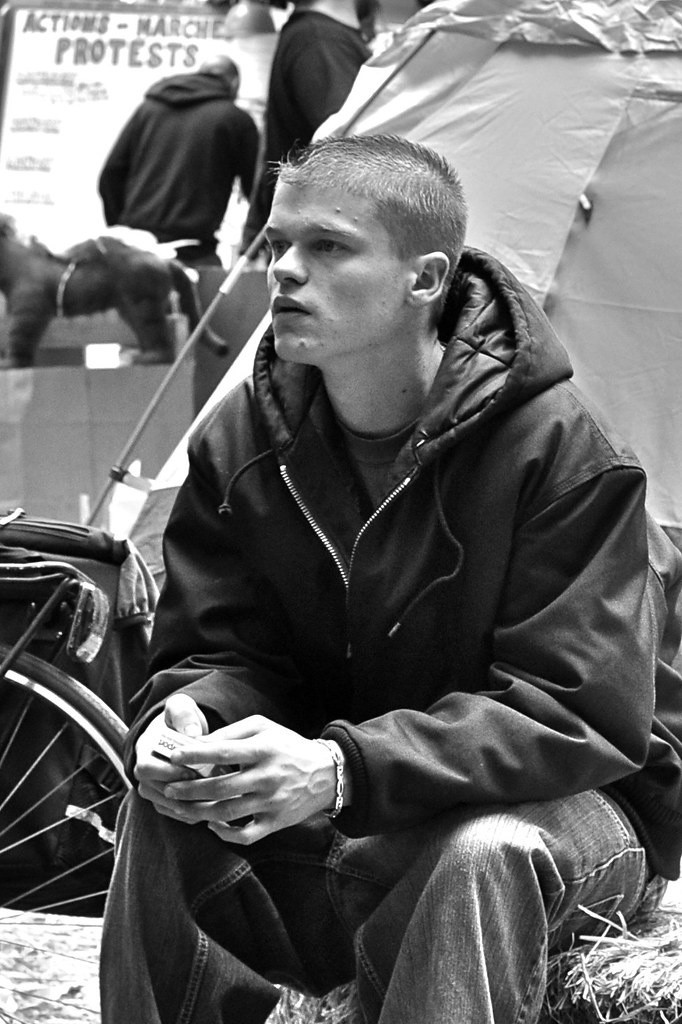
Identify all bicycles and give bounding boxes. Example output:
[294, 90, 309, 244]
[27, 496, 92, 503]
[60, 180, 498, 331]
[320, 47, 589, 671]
[0, 510, 218, 1024]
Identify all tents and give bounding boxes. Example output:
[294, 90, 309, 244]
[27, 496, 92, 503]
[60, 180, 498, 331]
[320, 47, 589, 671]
[127, 1, 681, 638]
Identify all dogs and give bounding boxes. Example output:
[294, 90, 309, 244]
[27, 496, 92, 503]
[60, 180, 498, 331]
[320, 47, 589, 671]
[1, 214, 230, 368]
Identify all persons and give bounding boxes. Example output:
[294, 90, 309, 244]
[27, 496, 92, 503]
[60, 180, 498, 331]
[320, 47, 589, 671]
[97, 134, 682, 1024]
[99, 54, 262, 271]
[237, 0, 378, 268]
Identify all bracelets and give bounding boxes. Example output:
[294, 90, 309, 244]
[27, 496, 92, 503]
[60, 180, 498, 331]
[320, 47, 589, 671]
[313, 737, 347, 819]
